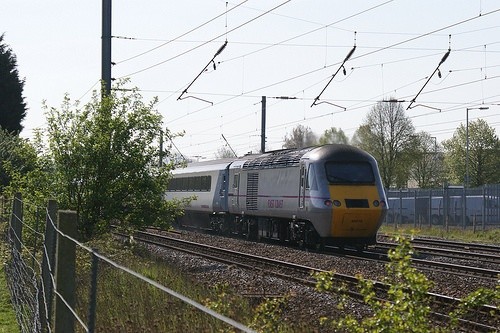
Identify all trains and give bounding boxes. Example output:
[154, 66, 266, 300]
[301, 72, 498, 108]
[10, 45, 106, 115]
[383, 194, 499, 226]
[53, 143, 390, 254]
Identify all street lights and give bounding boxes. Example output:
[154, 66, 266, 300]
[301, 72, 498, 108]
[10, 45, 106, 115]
[465, 106, 490, 189]
[406, 134, 438, 185]
